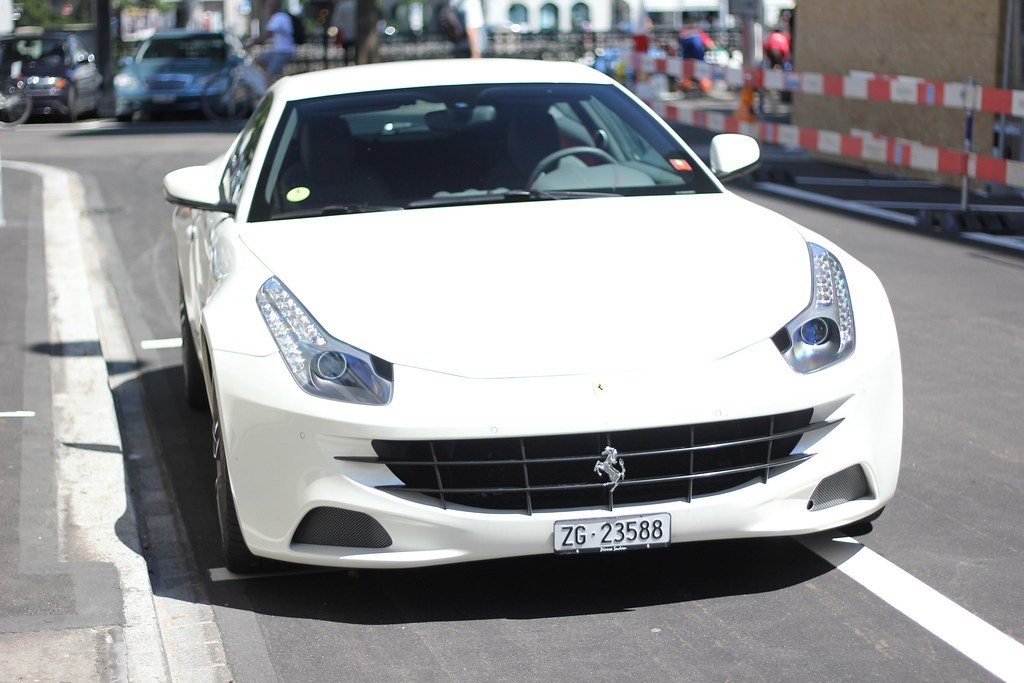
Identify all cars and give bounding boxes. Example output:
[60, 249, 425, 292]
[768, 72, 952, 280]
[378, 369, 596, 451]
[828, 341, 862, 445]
[163, 57, 905, 573]
[0, 31, 104, 124]
[113, 30, 253, 122]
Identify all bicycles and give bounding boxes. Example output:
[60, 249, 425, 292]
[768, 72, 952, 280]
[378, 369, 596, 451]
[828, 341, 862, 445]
[0, 80, 32, 127]
[201, 53, 284, 123]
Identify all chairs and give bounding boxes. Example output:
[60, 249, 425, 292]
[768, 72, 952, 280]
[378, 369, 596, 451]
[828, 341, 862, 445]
[278, 113, 578, 207]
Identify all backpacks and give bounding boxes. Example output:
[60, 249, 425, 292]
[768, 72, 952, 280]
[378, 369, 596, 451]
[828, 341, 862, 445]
[439, 0, 466, 43]
[278, 11, 304, 45]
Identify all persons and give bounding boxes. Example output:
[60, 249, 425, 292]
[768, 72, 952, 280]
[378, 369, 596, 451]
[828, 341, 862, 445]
[630, 21, 792, 96]
[243, 2, 300, 89]
